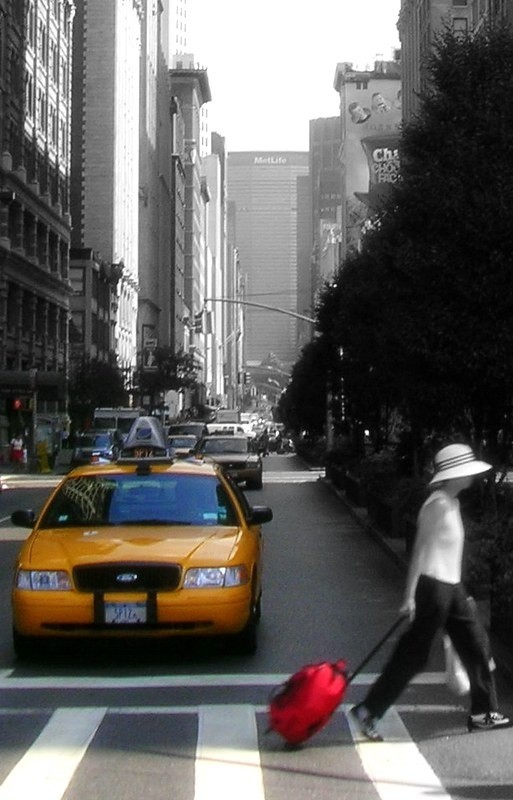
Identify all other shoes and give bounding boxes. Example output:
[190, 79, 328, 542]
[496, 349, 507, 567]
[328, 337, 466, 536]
[467, 711, 513, 732]
[348, 702, 385, 742]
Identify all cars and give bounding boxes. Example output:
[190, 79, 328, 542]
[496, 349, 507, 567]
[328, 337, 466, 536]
[10, 415, 275, 666]
[70, 406, 281, 491]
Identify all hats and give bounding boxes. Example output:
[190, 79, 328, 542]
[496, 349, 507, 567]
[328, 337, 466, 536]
[429, 444, 492, 484]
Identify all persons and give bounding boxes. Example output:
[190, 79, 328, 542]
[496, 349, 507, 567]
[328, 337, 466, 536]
[258, 426, 294, 458]
[348, 443, 513, 743]
[7, 426, 85, 473]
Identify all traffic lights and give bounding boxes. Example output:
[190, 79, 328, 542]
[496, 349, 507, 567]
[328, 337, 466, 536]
[244, 371, 251, 385]
[193, 311, 207, 335]
[13, 399, 22, 411]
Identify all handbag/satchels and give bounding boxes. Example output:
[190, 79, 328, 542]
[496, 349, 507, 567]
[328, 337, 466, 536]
[441, 596, 496, 696]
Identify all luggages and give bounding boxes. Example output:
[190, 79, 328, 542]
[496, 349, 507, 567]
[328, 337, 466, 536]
[268, 609, 413, 741]
